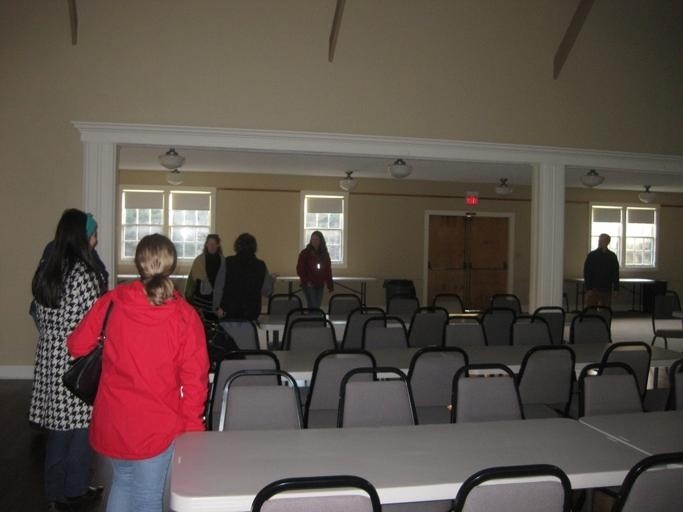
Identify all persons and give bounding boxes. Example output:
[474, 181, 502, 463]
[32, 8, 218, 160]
[584, 234, 619, 308]
[67, 233, 212, 512]
[185, 234, 224, 322]
[28, 209, 109, 512]
[297, 231, 334, 308]
[212, 233, 280, 320]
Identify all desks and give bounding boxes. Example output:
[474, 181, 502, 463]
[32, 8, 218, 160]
[164, 409, 681, 512]
[117, 273, 186, 295]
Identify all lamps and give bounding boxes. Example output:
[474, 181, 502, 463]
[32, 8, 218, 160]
[494, 177, 512, 194]
[637, 183, 656, 203]
[387, 158, 410, 179]
[337, 170, 358, 191]
[165, 168, 183, 185]
[579, 168, 603, 186]
[157, 147, 184, 169]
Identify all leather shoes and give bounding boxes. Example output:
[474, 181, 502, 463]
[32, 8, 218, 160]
[40, 484, 105, 506]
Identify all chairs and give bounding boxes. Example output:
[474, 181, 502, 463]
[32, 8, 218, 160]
[451, 464, 572, 512]
[577, 453, 680, 511]
[187, 278, 680, 432]
[250, 475, 382, 512]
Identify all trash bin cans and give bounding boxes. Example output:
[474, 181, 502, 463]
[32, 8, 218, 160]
[643, 281, 667, 313]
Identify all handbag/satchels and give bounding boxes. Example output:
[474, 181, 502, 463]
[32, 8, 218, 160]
[60, 298, 115, 408]
